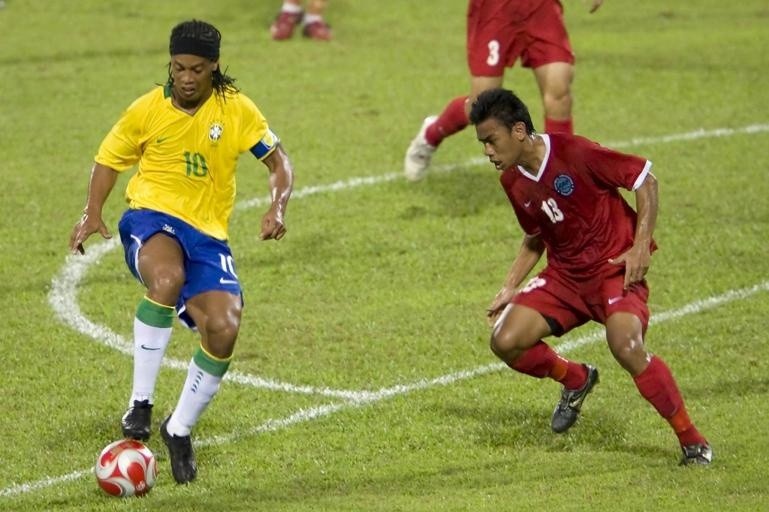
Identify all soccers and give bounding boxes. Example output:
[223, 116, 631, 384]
[95, 437, 159, 497]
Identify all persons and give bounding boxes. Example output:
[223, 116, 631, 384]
[468, 88, 714, 470]
[268, 0, 333, 41]
[67, 18, 297, 485]
[403, 0, 576, 186]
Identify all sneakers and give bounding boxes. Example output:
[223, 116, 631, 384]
[120, 398, 155, 442]
[158, 413, 198, 482]
[270, 9, 336, 41]
[404, 115, 440, 182]
[675, 435, 712, 467]
[551, 362, 600, 434]
